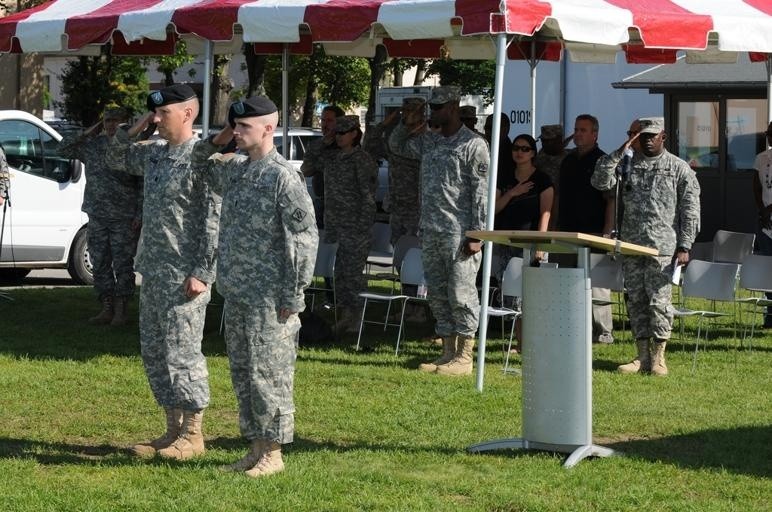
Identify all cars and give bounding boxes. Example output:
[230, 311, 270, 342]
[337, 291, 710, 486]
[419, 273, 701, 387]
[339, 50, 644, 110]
[189, 125, 203, 141]
[310, 101, 334, 124]
[46, 119, 85, 139]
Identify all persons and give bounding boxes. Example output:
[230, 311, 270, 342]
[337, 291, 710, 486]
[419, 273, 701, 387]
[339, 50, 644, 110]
[484, 112, 701, 376]
[299, 85, 490, 376]
[55, 85, 319, 478]
[752, 122, 772, 329]
[1, 145, 10, 205]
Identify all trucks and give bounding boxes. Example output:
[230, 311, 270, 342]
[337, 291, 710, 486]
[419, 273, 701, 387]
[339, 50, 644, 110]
[373, 85, 493, 137]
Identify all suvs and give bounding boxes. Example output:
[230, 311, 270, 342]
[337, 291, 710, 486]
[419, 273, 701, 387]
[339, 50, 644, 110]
[209, 127, 390, 219]
[0, 110, 97, 287]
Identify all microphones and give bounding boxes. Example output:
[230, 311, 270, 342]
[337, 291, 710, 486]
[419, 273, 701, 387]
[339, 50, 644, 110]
[623, 148, 633, 183]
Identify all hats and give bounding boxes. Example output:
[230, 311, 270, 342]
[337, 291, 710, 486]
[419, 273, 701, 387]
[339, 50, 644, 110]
[640, 117, 665, 134]
[103, 110, 121, 120]
[334, 115, 359, 132]
[396, 86, 476, 118]
[538, 124, 562, 139]
[228, 97, 278, 128]
[147, 84, 197, 113]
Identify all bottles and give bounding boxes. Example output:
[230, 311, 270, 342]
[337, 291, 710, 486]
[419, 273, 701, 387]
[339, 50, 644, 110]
[417, 272, 428, 301]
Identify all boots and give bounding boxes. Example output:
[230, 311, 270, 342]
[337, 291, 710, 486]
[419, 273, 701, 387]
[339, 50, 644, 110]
[617, 338, 667, 375]
[388, 304, 429, 324]
[332, 306, 365, 332]
[218, 439, 285, 478]
[418, 336, 476, 376]
[89, 296, 127, 326]
[130, 404, 205, 461]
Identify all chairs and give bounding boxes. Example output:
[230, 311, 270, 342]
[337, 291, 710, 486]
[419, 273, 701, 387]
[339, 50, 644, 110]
[206, 282, 226, 336]
[354, 230, 428, 361]
[368, 221, 395, 255]
[690, 242, 708, 260]
[586, 249, 625, 342]
[304, 242, 340, 331]
[475, 255, 559, 374]
[668, 260, 742, 377]
[735, 254, 771, 354]
[708, 230, 756, 266]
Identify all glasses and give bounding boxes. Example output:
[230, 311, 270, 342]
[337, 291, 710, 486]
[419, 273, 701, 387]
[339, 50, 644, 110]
[627, 131, 639, 135]
[335, 129, 353, 135]
[430, 103, 453, 110]
[512, 145, 533, 152]
